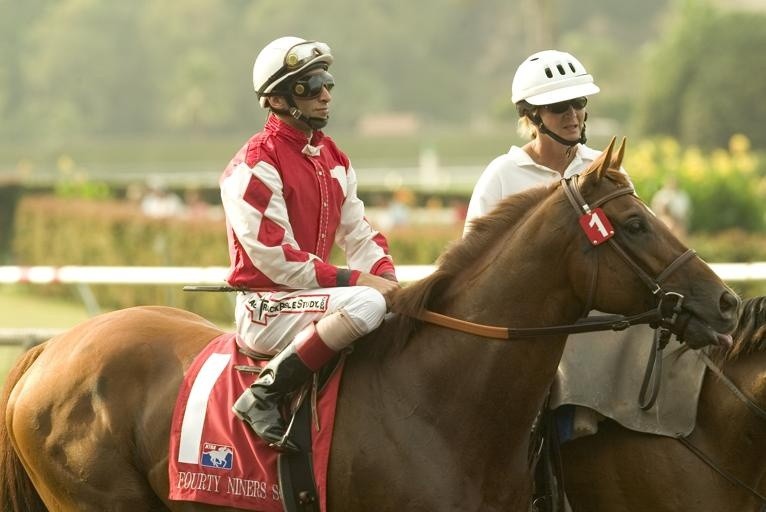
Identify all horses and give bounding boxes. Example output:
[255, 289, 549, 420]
[528, 297, 766, 512]
[0, 136, 742, 512]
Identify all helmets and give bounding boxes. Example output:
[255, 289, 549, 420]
[251, 35, 334, 102]
[509, 48, 601, 107]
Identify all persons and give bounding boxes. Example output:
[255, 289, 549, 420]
[463, 50, 636, 241]
[220, 35, 404, 454]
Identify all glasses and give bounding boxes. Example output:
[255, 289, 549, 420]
[547, 97, 591, 114]
[291, 73, 338, 95]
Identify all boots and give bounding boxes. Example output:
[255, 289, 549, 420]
[233, 309, 343, 457]
